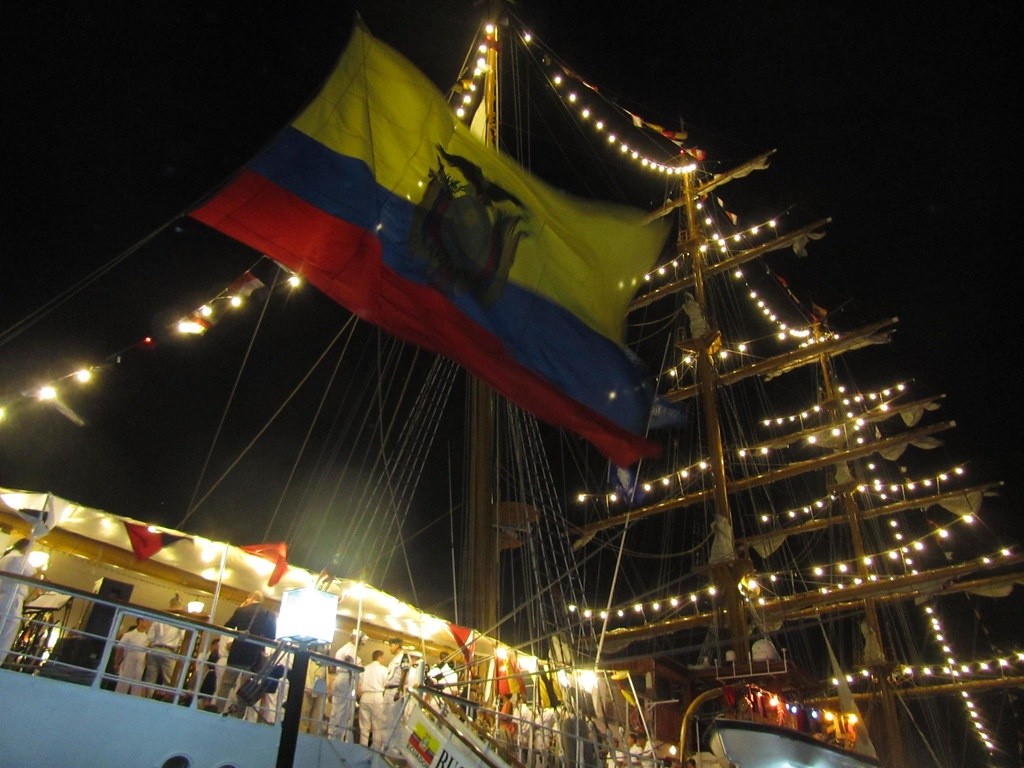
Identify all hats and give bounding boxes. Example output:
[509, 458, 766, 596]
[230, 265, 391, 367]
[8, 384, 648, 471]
[409, 651, 422, 658]
[352, 629, 366, 637]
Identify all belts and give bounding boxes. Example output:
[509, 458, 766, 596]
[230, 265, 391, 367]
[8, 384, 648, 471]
[386, 685, 399, 688]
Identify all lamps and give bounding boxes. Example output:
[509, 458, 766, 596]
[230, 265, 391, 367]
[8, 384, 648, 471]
[187, 594, 205, 613]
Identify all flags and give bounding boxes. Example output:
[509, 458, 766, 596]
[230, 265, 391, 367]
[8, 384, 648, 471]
[489, 641, 529, 699]
[180, 22, 676, 469]
[115, 519, 196, 564]
[531, 659, 565, 709]
[240, 541, 289, 586]
[445, 621, 476, 680]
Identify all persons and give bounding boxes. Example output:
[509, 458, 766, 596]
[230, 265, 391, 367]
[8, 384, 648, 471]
[0, 539, 665, 767]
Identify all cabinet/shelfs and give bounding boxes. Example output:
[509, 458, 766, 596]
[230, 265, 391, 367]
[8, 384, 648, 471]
[161, 608, 210, 704]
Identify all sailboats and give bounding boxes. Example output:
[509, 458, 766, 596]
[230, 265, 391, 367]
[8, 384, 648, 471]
[0, 0, 1024, 767]
